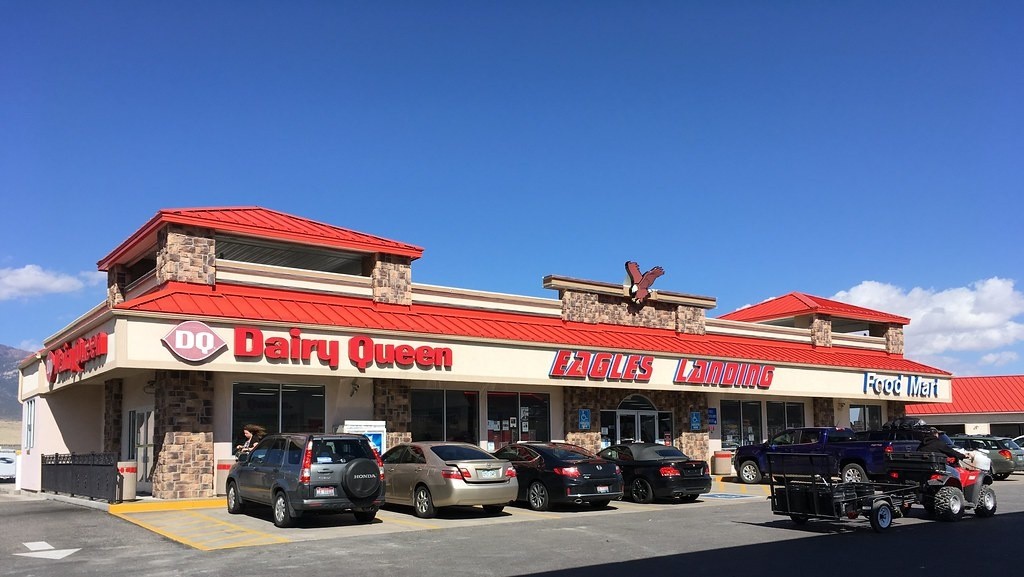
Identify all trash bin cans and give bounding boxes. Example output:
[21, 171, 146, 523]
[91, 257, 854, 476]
[714, 451, 732, 475]
[115, 461, 137, 501]
[217, 459, 233, 495]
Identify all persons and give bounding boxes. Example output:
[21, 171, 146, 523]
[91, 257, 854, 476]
[236, 423, 267, 453]
[916, 427, 969, 459]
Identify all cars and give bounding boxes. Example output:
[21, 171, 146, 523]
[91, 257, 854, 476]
[491, 440, 624, 512]
[380, 441, 519, 519]
[0, 449, 16, 483]
[595, 443, 712, 504]
[1004, 435, 1024, 450]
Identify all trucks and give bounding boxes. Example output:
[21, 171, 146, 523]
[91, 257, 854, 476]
[766, 445, 997, 532]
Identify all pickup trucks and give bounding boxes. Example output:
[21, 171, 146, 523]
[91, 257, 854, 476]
[733, 427, 920, 484]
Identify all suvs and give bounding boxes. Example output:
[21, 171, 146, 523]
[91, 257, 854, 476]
[950, 434, 1024, 481]
[225, 433, 386, 529]
